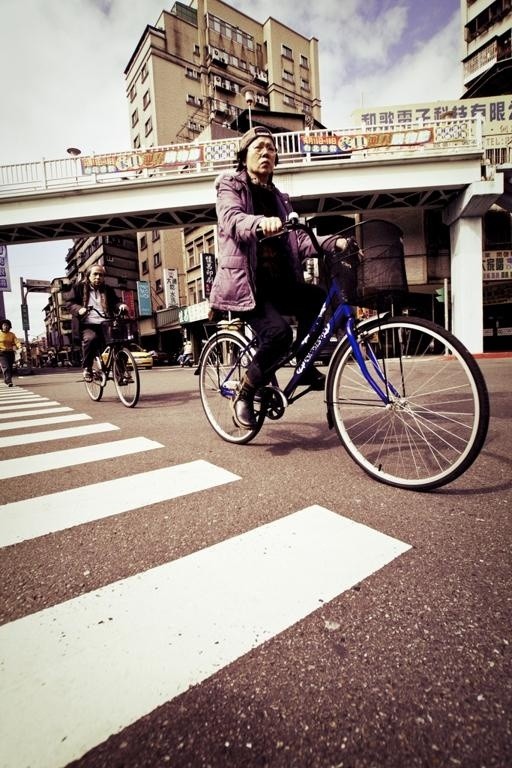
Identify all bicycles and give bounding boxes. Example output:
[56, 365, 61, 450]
[195, 209, 492, 493]
[78, 304, 143, 409]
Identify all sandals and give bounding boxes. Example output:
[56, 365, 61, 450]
[122, 377, 134, 383]
[83, 367, 93, 382]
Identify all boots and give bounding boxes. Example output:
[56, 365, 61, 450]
[230, 376, 259, 430]
[296, 355, 325, 387]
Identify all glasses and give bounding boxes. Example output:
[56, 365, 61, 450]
[246, 144, 280, 157]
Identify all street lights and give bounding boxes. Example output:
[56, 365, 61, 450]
[65, 147, 83, 187]
[239, 82, 262, 132]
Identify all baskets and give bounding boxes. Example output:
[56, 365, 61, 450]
[320, 218, 411, 313]
[101, 317, 139, 349]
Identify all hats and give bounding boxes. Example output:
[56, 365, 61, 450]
[236, 125, 276, 152]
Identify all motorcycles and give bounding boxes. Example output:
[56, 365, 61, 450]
[173, 347, 195, 368]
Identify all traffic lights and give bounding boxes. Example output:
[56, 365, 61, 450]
[434, 286, 444, 304]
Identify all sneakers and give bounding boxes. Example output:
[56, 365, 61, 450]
[4, 376, 13, 387]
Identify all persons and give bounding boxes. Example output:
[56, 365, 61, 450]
[66, 263, 132, 386]
[0, 318, 22, 386]
[209, 126, 364, 429]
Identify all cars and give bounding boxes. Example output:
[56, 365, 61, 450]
[147, 347, 175, 367]
[12, 349, 81, 370]
[98, 341, 154, 371]
